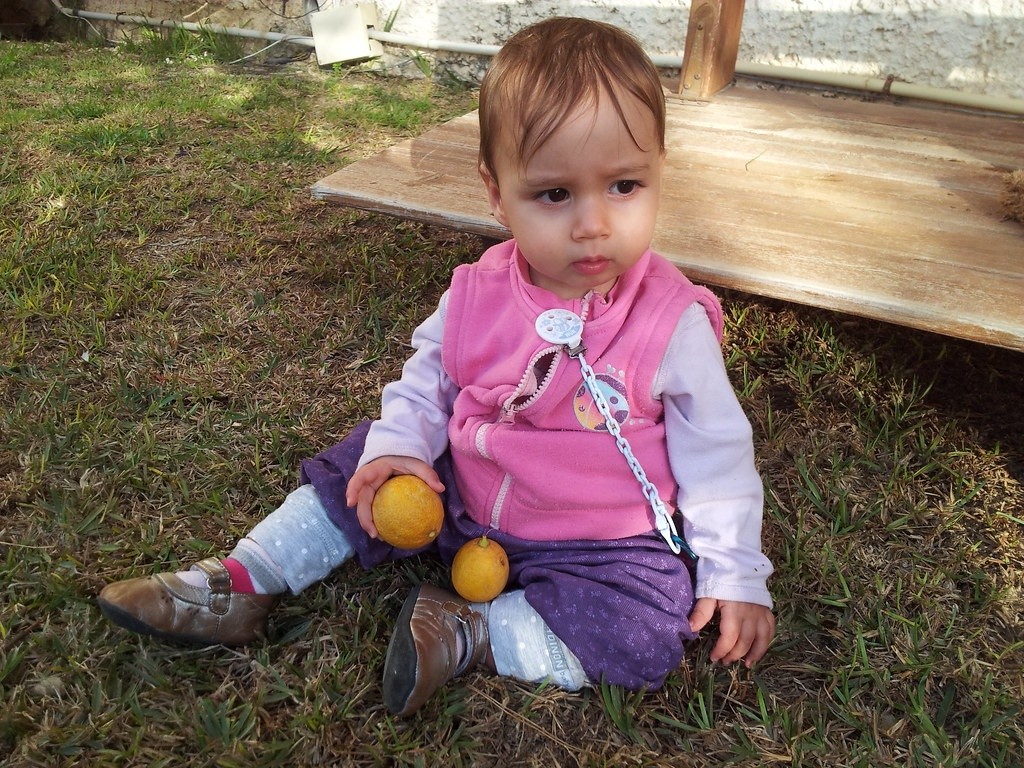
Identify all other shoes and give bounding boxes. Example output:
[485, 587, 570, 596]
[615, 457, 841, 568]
[97, 556, 277, 649]
[385, 582, 489, 716]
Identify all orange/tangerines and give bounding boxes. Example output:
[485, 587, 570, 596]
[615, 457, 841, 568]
[373, 475, 444, 549]
[451, 535, 509, 603]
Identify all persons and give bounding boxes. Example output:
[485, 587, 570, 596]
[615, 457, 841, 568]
[93, 16, 775, 717]
